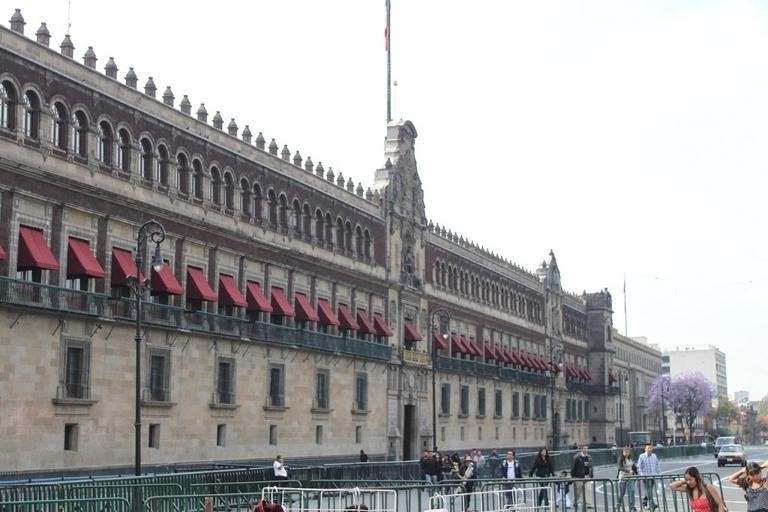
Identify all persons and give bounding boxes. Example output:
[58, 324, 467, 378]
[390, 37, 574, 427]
[529, 445, 593, 509]
[729, 460, 768, 511]
[273, 455, 295, 502]
[360, 450, 369, 463]
[615, 443, 664, 512]
[668, 467, 725, 511]
[419, 442, 525, 509]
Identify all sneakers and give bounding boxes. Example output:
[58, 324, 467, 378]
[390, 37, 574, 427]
[555, 503, 593, 509]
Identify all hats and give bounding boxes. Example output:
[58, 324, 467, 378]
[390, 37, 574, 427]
[465, 455, 473, 461]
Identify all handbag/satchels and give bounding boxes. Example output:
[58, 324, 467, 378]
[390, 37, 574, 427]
[632, 465, 638, 476]
[704, 483, 728, 512]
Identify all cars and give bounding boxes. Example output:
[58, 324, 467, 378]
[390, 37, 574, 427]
[715, 443, 748, 469]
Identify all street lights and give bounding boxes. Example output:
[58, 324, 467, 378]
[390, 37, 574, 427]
[549, 339, 564, 450]
[430, 305, 451, 454]
[660, 377, 671, 447]
[616, 366, 630, 448]
[123, 217, 168, 511]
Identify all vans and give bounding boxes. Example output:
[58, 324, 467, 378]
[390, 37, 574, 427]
[713, 435, 738, 458]
